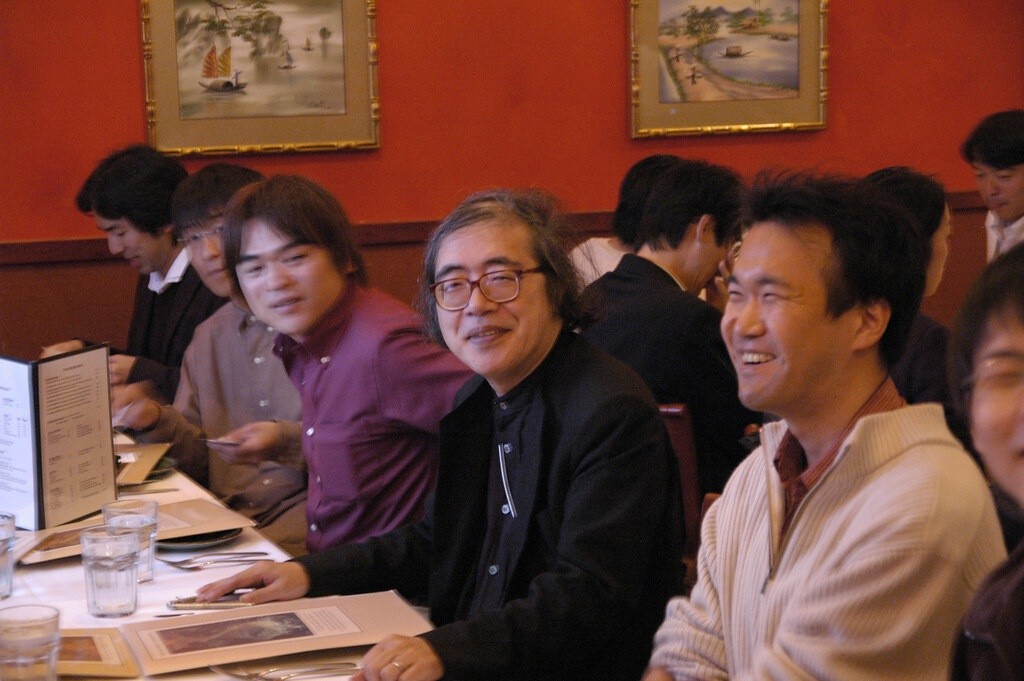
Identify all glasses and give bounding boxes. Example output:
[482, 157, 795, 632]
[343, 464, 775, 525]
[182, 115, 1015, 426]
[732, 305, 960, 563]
[967, 357, 1024, 408]
[427, 268, 543, 311]
[178, 224, 223, 251]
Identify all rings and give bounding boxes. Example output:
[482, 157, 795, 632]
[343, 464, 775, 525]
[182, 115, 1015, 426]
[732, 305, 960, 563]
[392, 661, 404, 672]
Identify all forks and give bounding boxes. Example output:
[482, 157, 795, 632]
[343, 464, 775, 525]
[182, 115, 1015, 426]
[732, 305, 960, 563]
[209, 662, 363, 680]
[155, 551, 275, 571]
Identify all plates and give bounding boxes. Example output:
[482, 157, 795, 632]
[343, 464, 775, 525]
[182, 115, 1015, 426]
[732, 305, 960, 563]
[156, 527, 244, 549]
[149, 457, 178, 476]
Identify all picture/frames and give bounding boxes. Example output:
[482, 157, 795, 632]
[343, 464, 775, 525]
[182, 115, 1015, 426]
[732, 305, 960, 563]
[137, 0, 380, 158]
[629, 0, 829, 139]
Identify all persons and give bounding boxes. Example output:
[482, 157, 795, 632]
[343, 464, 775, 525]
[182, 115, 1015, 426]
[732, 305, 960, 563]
[569, 153, 761, 505]
[860, 166, 971, 442]
[959, 109, 1024, 261]
[943, 240, 1024, 681]
[108, 162, 306, 553]
[643, 168, 1008, 681]
[36, 144, 231, 414]
[216, 173, 475, 555]
[199, 187, 689, 681]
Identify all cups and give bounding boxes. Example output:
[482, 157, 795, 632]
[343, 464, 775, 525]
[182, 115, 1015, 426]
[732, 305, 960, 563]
[0, 511, 15, 600]
[80, 526, 138, 616]
[102, 500, 157, 583]
[1, 606, 59, 681]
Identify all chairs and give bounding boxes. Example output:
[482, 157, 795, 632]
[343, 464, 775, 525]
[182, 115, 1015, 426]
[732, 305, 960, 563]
[656, 403, 698, 553]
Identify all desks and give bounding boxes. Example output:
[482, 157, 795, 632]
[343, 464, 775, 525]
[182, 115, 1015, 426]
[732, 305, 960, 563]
[0, 426, 383, 681]
